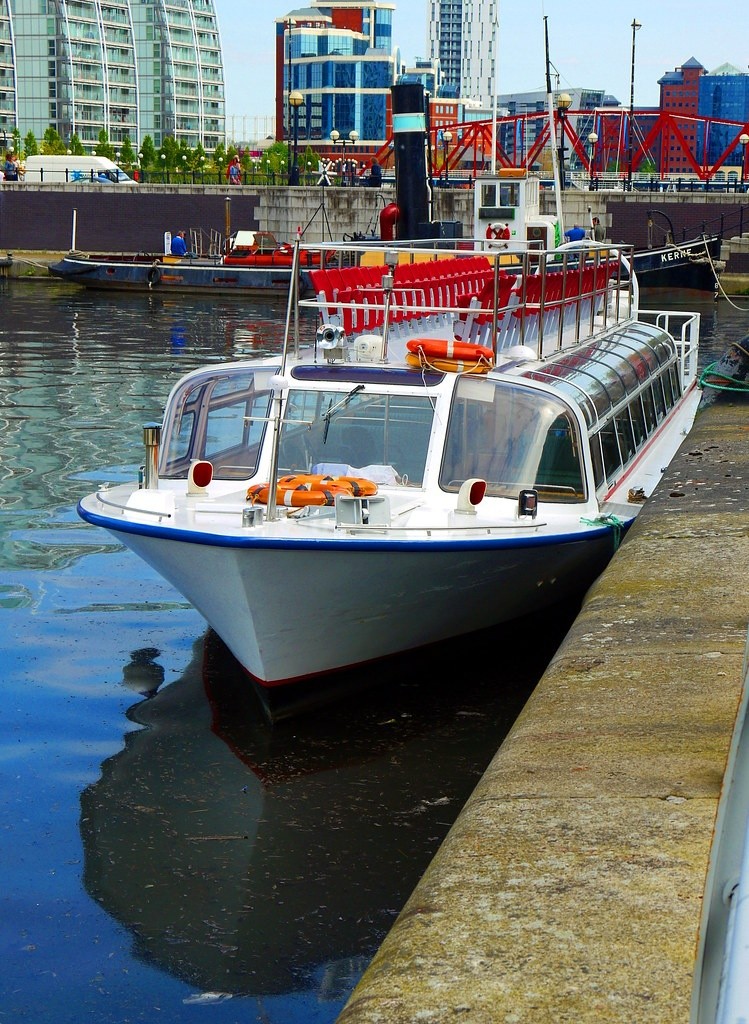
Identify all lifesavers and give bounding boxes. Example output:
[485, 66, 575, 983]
[486, 222, 510, 248]
[278, 244, 295, 253]
[406, 353, 491, 375]
[405, 338, 492, 360]
[281, 478, 378, 495]
[248, 481, 354, 507]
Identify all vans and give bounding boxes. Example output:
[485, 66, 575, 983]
[24, 155, 138, 184]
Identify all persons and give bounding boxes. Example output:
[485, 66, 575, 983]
[97, 172, 107, 182]
[226, 154, 241, 185]
[591, 217, 604, 243]
[367, 157, 382, 187]
[171, 231, 187, 256]
[563, 223, 585, 258]
[4, 154, 19, 181]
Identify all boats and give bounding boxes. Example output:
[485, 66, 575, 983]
[46, 74, 721, 320]
[77, 14, 705, 692]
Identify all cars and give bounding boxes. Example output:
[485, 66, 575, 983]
[70, 176, 115, 184]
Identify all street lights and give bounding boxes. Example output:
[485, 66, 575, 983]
[588, 133, 597, 192]
[627, 17, 643, 192]
[442, 131, 452, 188]
[289, 91, 304, 186]
[330, 128, 359, 187]
[558, 92, 573, 192]
[739, 134, 749, 193]
[7, 146, 313, 186]
